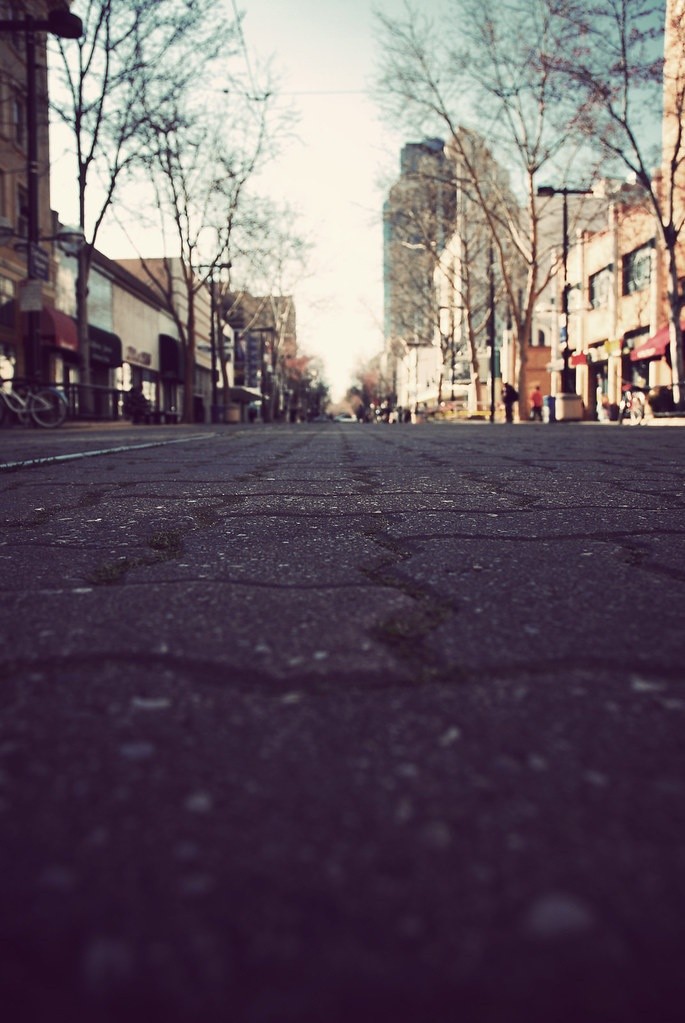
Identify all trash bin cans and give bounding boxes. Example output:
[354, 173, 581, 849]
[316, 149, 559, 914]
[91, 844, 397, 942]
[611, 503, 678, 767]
[543, 393, 583, 423]
[213, 404, 240, 424]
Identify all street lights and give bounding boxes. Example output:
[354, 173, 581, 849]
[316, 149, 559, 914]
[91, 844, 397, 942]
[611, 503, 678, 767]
[536, 186, 595, 395]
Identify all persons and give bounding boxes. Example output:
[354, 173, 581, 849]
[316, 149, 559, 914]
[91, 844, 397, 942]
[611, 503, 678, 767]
[355, 400, 430, 422]
[501, 382, 520, 424]
[618, 383, 646, 427]
[528, 384, 544, 421]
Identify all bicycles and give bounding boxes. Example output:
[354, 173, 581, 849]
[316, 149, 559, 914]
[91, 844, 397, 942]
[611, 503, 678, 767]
[0, 368, 70, 431]
[618, 384, 646, 427]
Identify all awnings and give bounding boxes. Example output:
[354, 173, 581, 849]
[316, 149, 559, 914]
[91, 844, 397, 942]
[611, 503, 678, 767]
[630, 321, 685, 361]
[37, 306, 123, 369]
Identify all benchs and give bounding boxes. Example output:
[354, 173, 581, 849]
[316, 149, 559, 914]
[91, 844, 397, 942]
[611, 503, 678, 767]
[132, 412, 179, 426]
